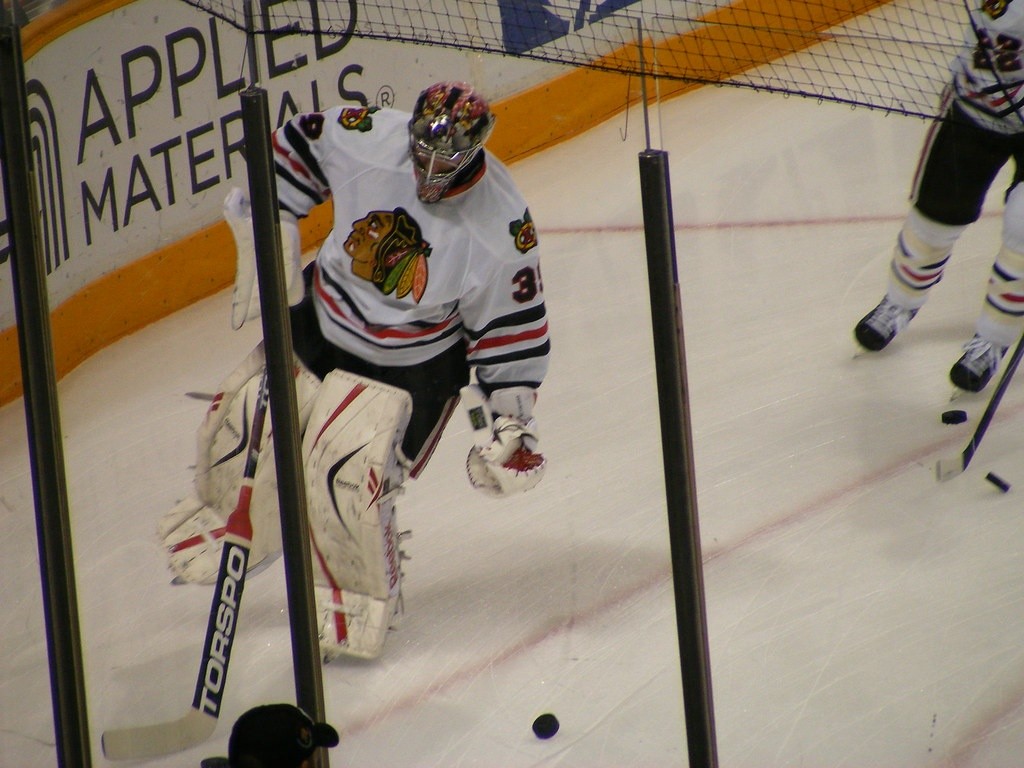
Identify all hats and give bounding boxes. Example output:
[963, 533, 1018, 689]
[229, 703, 340, 768]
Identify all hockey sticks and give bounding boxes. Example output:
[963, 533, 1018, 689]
[985, 472, 1011, 493]
[101, 367, 270, 760]
[936, 333, 1024, 482]
[941, 410, 968, 424]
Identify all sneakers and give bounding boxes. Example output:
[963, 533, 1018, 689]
[854, 296, 918, 358]
[948, 332, 1010, 404]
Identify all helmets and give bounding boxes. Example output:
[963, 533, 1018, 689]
[410, 78, 496, 202]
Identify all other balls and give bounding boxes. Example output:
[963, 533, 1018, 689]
[532, 714, 560, 739]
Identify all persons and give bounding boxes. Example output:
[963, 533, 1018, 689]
[200, 703, 339, 768]
[854, 0, 1024, 401]
[158, 83, 550, 663]
